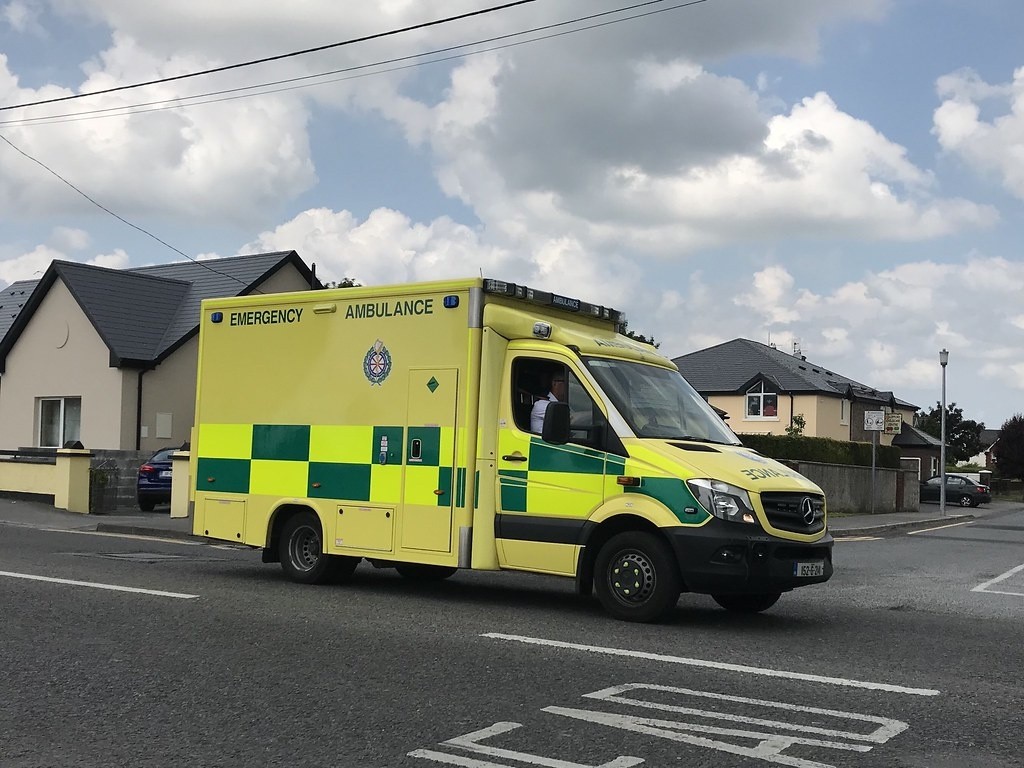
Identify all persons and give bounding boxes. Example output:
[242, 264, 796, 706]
[530, 367, 594, 439]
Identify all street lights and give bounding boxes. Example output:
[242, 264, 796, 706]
[939, 349, 949, 515]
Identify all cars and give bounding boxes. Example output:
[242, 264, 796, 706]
[137, 447, 184, 510]
[920, 473, 994, 508]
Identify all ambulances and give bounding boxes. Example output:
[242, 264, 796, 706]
[185, 276, 836, 620]
